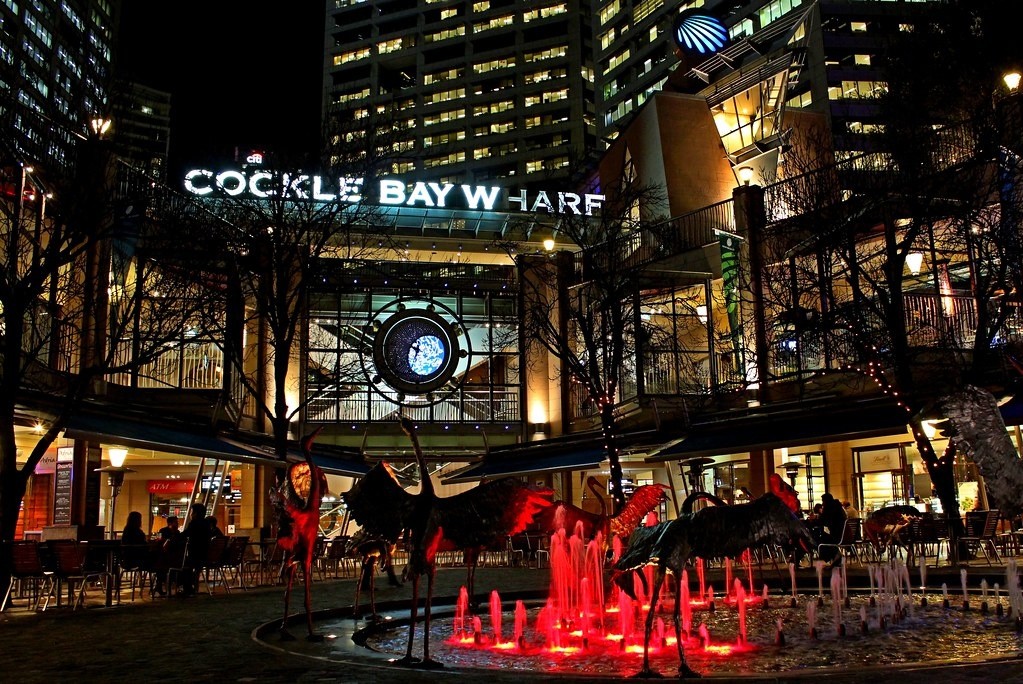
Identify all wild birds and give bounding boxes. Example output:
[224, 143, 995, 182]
[609, 491, 817, 676]
[340, 418, 556, 665]
[863, 385, 1023, 575]
[269, 425, 329, 640]
[538, 476, 672, 579]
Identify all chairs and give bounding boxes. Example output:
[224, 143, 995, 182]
[0, 524, 549, 615]
[816, 509, 1012, 568]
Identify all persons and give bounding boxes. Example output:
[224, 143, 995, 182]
[843, 500, 859, 519]
[928, 489, 943, 513]
[163, 503, 212, 592]
[914, 494, 926, 512]
[206, 515, 224, 543]
[813, 503, 822, 515]
[817, 494, 853, 545]
[121, 512, 166, 595]
[159, 516, 181, 539]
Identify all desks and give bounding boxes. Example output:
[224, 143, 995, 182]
[324, 539, 351, 543]
[1009, 531, 1023, 555]
[519, 535, 548, 568]
